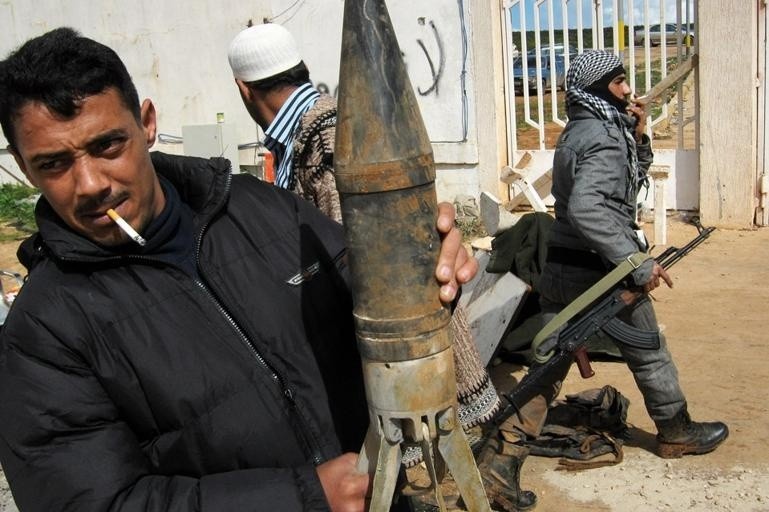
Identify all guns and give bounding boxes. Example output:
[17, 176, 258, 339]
[482, 217, 715, 437]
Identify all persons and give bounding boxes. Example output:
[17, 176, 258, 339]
[0, 26, 477, 512]
[479, 50, 730, 511]
[227, 22, 503, 468]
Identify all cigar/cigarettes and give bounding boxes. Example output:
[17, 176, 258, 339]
[631, 95, 649, 102]
[107, 208, 150, 252]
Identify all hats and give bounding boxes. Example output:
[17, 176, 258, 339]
[229, 24, 302, 81]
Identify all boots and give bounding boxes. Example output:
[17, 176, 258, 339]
[655, 406, 728, 459]
[475, 431, 537, 512]
